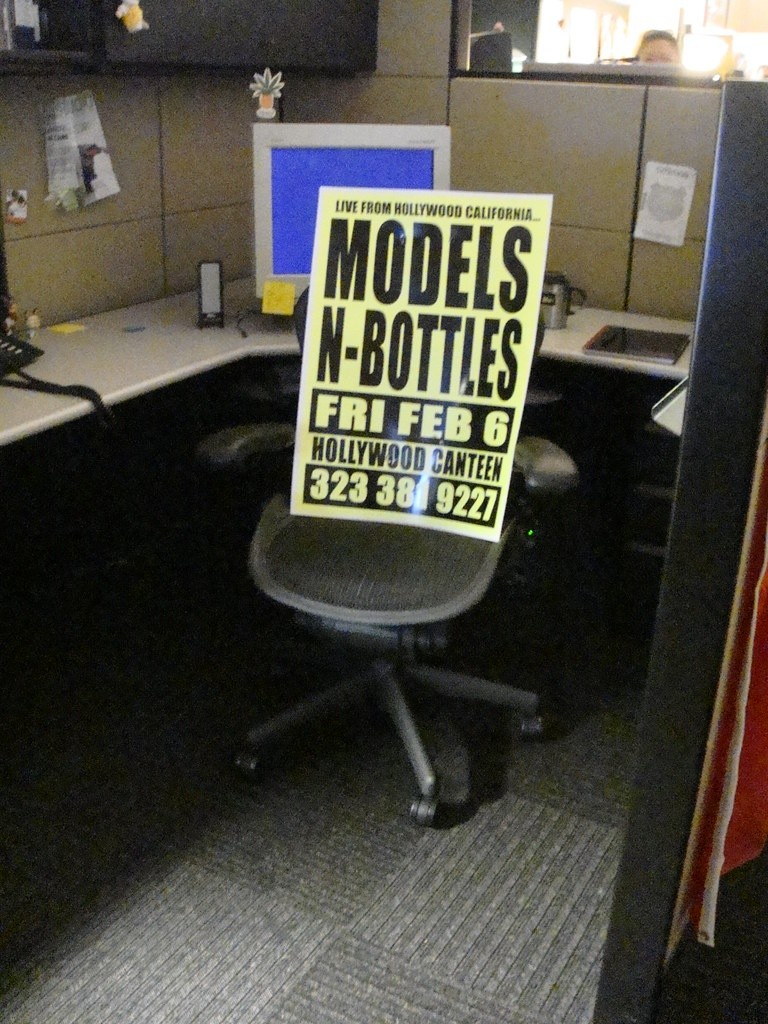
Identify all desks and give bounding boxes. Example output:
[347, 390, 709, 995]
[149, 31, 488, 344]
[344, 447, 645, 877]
[0, 276, 696, 447]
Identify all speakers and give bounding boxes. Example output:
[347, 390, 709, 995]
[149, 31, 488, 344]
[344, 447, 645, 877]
[196, 259, 224, 330]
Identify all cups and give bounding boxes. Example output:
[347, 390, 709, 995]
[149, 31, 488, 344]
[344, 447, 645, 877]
[539, 271, 588, 329]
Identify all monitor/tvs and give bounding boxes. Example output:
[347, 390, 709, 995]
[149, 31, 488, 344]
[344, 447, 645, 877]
[253, 120, 452, 298]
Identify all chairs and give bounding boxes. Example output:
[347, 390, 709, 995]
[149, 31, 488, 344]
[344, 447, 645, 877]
[190, 288, 579, 827]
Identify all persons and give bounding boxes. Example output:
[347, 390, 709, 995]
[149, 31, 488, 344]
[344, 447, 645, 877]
[636, 32, 679, 64]
[81, 144, 99, 195]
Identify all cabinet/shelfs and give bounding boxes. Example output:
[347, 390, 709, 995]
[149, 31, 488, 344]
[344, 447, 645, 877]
[0, 0, 380, 79]
